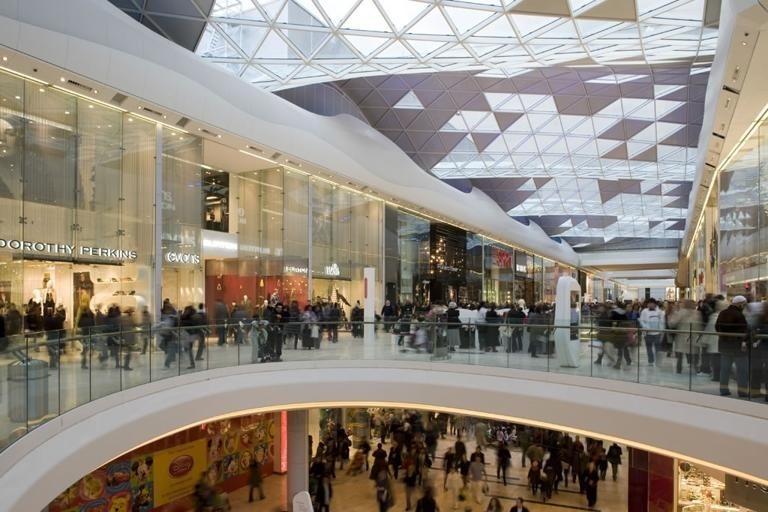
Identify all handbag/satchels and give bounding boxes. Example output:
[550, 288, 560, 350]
[498, 320, 515, 338]
[626, 326, 640, 348]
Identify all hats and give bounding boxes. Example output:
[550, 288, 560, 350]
[448, 301, 458, 308]
[732, 295, 746, 304]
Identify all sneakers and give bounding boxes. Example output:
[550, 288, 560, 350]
[697, 369, 712, 377]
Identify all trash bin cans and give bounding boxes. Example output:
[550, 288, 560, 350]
[7, 359, 48, 423]
